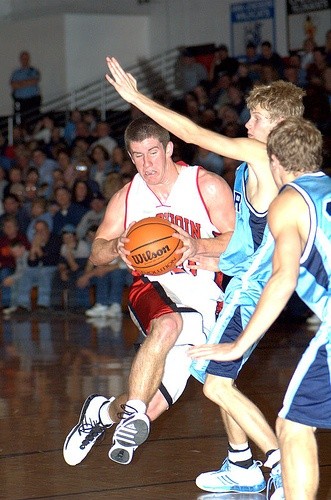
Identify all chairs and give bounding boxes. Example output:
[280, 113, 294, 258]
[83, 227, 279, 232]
[0, 44, 330, 308]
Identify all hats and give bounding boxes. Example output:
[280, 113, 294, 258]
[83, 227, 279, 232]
[57, 223, 77, 233]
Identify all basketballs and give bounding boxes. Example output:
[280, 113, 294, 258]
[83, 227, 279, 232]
[124, 217, 183, 277]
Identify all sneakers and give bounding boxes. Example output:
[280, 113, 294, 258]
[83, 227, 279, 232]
[195, 448, 286, 500]
[84, 302, 123, 318]
[108, 412, 150, 465]
[62, 393, 115, 466]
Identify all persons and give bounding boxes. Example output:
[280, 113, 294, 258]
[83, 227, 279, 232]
[9, 50, 43, 136]
[103, 55, 305, 500]
[60, 115, 238, 468]
[151, 9, 331, 192]
[1, 103, 150, 321]
[0, 316, 150, 438]
[184, 112, 331, 500]
